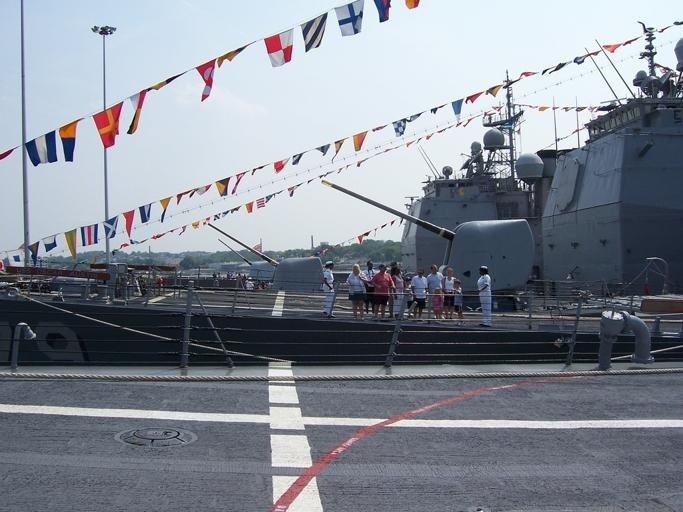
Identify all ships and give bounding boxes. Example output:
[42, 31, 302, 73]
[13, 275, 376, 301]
[0, 21, 682, 366]
[93, 238, 283, 289]
[4, 221, 380, 298]
[0, 68, 581, 303]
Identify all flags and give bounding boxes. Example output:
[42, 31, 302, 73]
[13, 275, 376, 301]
[1, 0, 681, 271]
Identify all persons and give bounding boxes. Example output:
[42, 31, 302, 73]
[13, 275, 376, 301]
[343, 260, 465, 326]
[211, 271, 270, 290]
[474, 266, 491, 327]
[323, 260, 335, 318]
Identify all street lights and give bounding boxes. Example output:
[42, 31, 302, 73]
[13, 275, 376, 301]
[89, 23, 116, 277]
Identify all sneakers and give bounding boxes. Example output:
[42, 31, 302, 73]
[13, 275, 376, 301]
[323, 313, 492, 327]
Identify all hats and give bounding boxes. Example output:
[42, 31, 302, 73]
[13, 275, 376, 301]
[418, 267, 424, 274]
[480, 266, 488, 269]
[406, 276, 411, 281]
[390, 262, 396, 266]
[325, 261, 332, 265]
